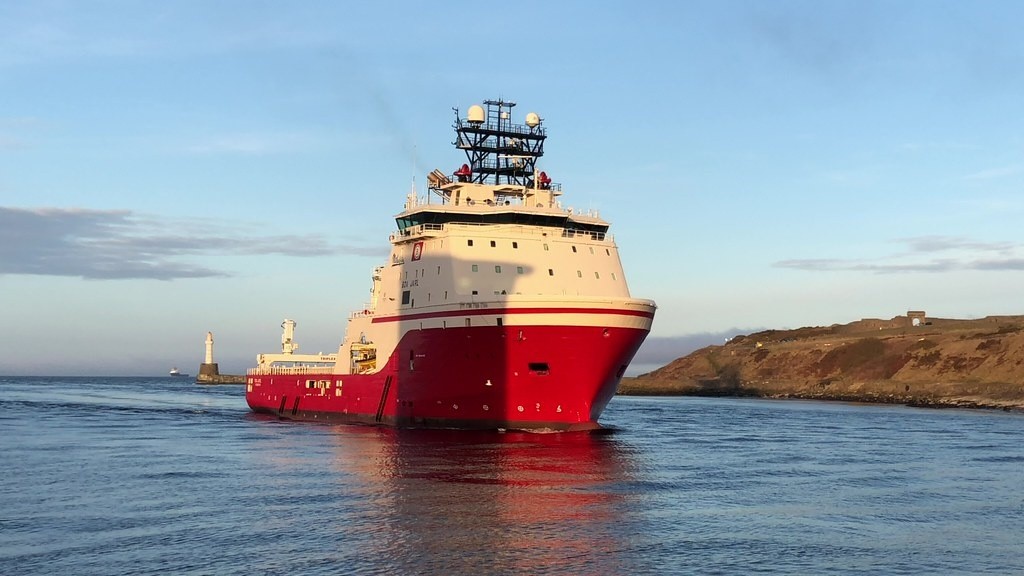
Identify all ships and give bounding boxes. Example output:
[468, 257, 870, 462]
[169, 368, 189, 377]
[245, 94, 657, 430]
